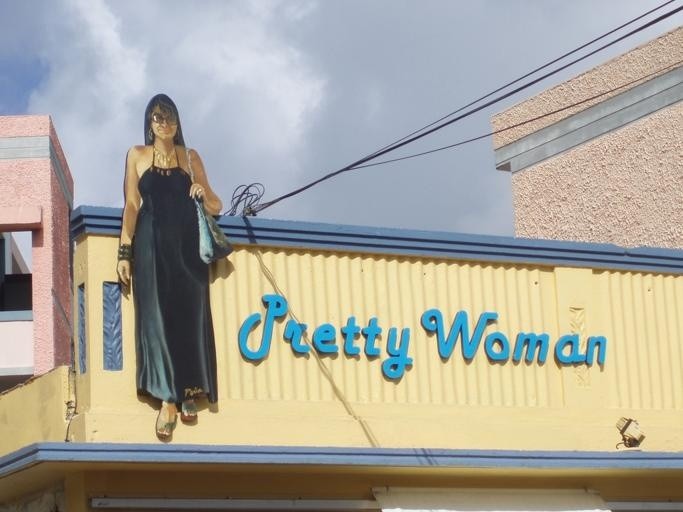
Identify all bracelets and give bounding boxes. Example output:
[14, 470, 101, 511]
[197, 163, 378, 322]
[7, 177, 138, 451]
[118, 243, 133, 260]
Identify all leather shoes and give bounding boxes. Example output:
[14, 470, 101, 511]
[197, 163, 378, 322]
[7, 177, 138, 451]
[180, 400, 198, 422]
[155, 414, 178, 442]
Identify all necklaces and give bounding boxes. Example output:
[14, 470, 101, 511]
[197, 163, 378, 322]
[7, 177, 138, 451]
[151, 144, 176, 176]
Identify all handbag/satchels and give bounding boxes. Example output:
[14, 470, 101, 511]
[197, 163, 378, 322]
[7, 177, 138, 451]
[195, 200, 233, 264]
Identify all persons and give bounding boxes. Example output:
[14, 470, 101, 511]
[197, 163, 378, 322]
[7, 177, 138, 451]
[116, 94, 223, 438]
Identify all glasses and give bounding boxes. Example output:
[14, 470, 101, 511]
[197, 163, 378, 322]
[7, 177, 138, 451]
[152, 111, 177, 126]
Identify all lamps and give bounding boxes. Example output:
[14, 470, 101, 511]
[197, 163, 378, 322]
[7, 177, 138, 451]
[615, 417, 646, 449]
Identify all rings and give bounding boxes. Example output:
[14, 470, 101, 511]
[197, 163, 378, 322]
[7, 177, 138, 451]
[198, 188, 202, 192]
